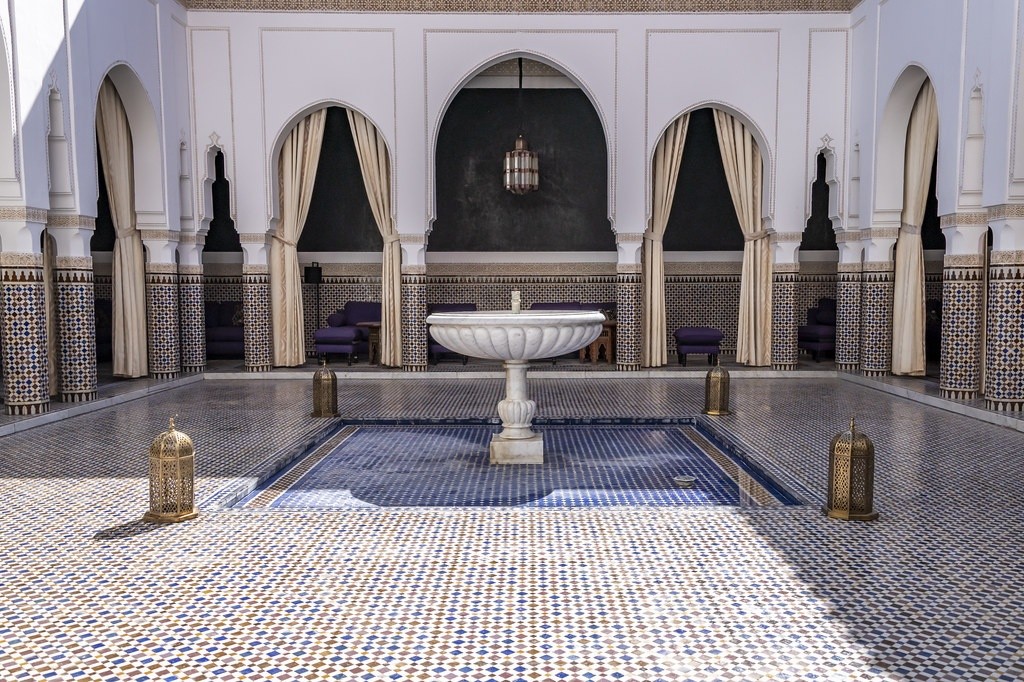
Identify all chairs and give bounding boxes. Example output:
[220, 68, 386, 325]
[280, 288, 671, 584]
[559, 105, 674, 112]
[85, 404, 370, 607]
[315, 301, 476, 366]
[205, 302, 245, 355]
[798, 298, 836, 362]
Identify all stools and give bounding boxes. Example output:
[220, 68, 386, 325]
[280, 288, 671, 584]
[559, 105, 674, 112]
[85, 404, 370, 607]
[673, 327, 723, 366]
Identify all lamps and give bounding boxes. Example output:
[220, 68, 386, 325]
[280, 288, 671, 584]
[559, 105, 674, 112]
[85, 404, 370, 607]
[304, 262, 322, 364]
[502, 57, 539, 195]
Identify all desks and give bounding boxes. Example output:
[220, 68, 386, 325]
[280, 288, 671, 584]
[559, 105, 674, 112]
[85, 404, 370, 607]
[578, 320, 617, 365]
[356, 322, 381, 365]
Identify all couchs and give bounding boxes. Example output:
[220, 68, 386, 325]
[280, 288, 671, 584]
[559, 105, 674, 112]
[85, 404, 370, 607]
[528, 302, 618, 365]
